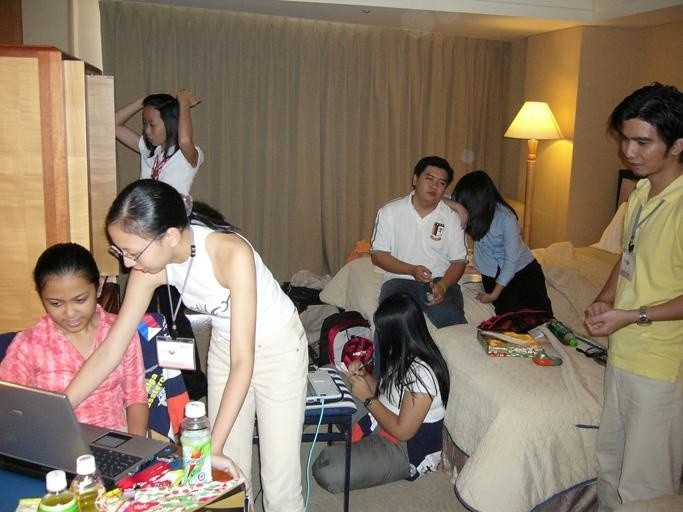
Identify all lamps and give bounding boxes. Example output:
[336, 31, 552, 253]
[502, 98, 565, 252]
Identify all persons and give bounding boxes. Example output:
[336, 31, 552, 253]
[367, 155, 470, 329]
[0, 243, 149, 441]
[114, 89, 208, 201]
[62, 178, 310, 511]
[310, 290, 450, 493]
[440, 168, 556, 319]
[578, 81, 682, 511]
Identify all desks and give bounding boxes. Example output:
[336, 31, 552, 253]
[0, 464, 245, 512]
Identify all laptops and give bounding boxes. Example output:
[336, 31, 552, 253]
[305, 370, 341, 405]
[0, 380, 177, 492]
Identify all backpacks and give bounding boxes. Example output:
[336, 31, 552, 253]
[320, 310, 374, 378]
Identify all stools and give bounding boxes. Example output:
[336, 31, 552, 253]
[246, 365, 357, 512]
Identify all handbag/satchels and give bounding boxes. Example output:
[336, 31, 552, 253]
[477, 307, 549, 333]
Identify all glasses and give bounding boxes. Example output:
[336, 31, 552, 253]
[109, 232, 156, 261]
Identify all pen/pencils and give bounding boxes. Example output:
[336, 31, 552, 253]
[353, 358, 373, 377]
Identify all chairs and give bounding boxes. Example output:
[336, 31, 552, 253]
[0, 310, 193, 449]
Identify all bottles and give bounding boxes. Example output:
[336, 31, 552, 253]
[545, 317, 577, 347]
[35, 467, 79, 511]
[178, 400, 214, 485]
[68, 454, 107, 511]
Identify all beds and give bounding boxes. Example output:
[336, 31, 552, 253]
[315, 240, 623, 329]
[422, 315, 609, 512]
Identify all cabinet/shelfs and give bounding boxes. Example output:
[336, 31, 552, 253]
[0, 44, 123, 334]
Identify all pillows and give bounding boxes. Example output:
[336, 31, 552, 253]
[586, 201, 632, 256]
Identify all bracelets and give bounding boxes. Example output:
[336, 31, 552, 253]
[436, 281, 446, 292]
[636, 303, 652, 327]
[363, 396, 378, 408]
